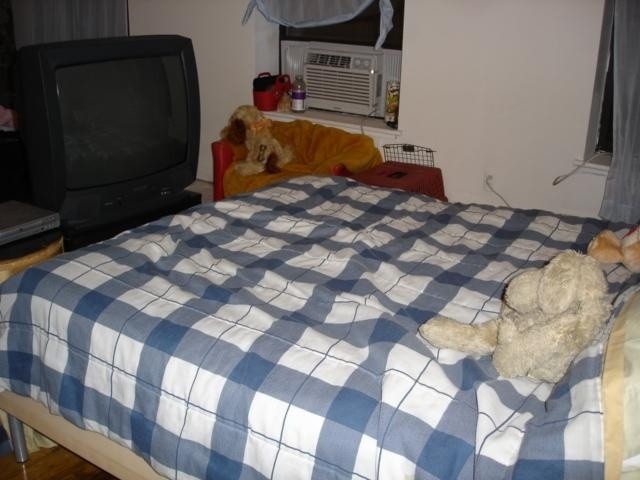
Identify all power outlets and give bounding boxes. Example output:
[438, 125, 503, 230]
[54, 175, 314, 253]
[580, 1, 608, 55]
[479, 172, 494, 192]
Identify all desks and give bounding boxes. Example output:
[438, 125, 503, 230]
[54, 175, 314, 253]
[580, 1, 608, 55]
[43, 190, 206, 248]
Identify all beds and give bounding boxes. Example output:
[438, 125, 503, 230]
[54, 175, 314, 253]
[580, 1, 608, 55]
[0, 171, 640, 479]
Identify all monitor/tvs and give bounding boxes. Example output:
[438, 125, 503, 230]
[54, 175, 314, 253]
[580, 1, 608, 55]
[12, 34, 201, 238]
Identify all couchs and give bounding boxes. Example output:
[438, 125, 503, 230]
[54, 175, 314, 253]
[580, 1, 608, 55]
[211, 119, 371, 201]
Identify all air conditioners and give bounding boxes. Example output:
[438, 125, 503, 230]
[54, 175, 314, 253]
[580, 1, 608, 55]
[300, 41, 381, 119]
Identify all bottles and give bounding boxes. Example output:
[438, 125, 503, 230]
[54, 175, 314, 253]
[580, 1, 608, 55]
[384, 79, 399, 125]
[290, 76, 306, 113]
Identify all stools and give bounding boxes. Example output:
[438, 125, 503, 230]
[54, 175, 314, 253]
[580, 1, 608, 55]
[1, 238, 65, 289]
[351, 158, 447, 202]
